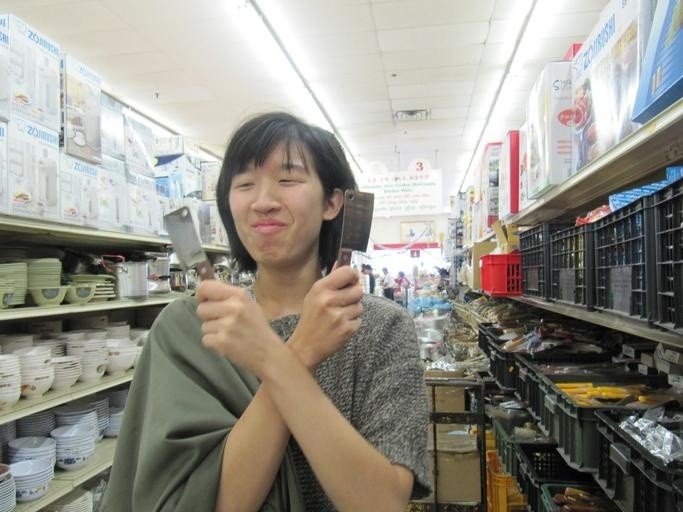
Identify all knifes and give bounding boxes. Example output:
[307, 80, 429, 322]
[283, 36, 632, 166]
[336, 189, 374, 270]
[162, 206, 216, 281]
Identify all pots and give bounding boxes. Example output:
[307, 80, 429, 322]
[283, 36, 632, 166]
[109, 261, 149, 298]
[169, 270, 189, 293]
[142, 251, 172, 294]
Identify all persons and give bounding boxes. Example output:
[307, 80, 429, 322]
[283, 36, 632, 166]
[393, 270, 411, 305]
[356, 263, 373, 295]
[361, 264, 376, 295]
[380, 266, 394, 301]
[94, 106, 438, 512]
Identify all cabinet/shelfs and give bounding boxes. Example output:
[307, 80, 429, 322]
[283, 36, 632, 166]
[0, 208, 232, 511]
[445, 97, 683, 512]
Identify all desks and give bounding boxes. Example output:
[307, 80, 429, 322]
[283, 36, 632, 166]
[409, 375, 487, 510]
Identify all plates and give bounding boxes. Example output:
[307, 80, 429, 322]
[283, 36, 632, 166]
[42, 488, 94, 512]
[66, 274, 116, 302]
[0, 258, 62, 308]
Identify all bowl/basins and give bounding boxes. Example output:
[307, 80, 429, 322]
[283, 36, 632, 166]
[0, 388, 129, 512]
[27, 286, 96, 308]
[1, 287, 14, 310]
[0, 315, 150, 411]
